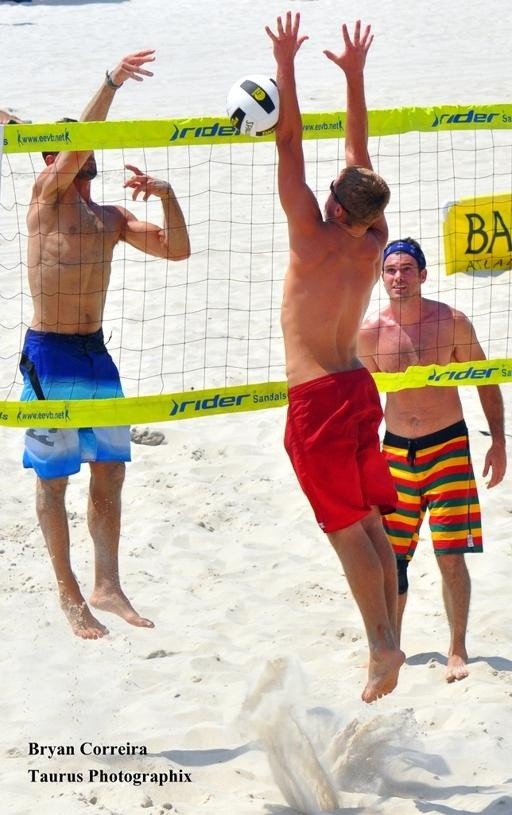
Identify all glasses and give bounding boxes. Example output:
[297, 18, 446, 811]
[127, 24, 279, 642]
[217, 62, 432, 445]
[330, 180, 350, 215]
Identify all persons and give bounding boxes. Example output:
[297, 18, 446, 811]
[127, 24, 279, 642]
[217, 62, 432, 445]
[22, 47, 193, 640]
[264, 7, 404, 703]
[356, 236, 506, 682]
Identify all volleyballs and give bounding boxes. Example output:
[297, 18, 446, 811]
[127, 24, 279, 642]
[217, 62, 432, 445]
[226, 73, 280, 134]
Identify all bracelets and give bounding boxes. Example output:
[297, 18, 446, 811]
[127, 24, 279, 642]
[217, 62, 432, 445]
[105, 68, 124, 89]
